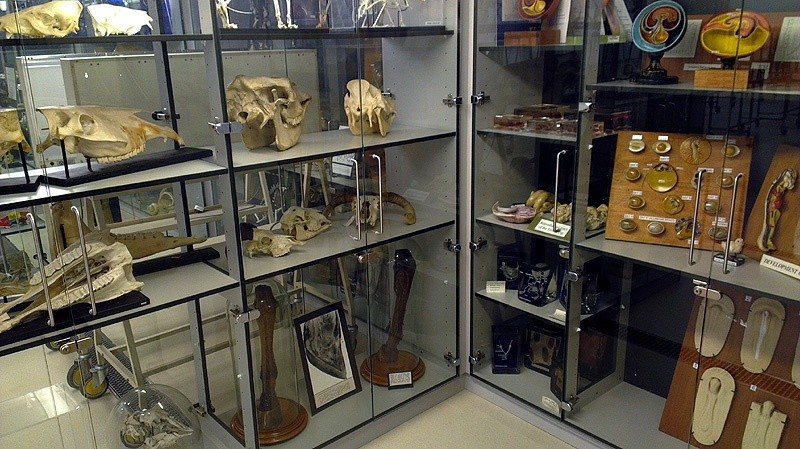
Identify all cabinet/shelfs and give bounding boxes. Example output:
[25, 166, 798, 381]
[0, 0, 800, 449]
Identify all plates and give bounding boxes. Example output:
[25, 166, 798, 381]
[515, 1, 561, 24]
[631, 0, 688, 53]
[702, 11, 772, 58]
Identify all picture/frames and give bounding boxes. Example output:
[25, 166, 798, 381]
[294, 300, 363, 417]
